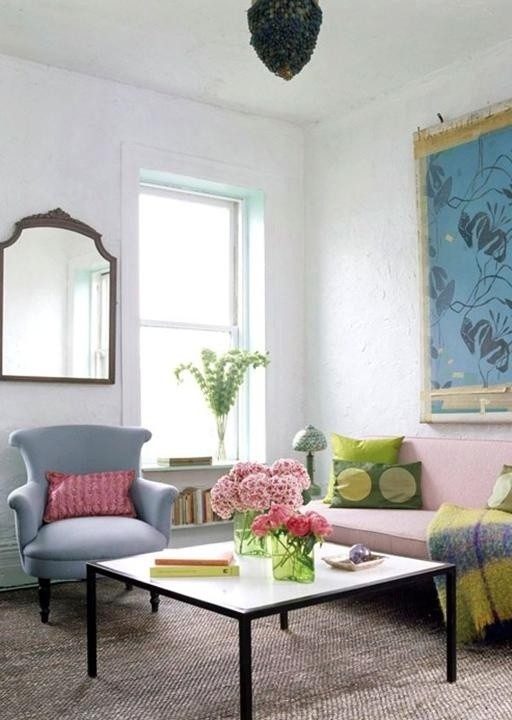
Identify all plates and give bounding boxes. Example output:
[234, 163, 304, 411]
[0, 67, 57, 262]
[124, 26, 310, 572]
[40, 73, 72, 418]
[321, 555, 387, 573]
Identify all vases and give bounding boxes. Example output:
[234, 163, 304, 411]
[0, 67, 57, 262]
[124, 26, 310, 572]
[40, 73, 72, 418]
[271, 528, 315, 584]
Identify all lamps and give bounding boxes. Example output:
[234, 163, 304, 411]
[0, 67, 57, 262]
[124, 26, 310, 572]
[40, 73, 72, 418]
[291, 424, 327, 495]
[247, 0, 323, 81]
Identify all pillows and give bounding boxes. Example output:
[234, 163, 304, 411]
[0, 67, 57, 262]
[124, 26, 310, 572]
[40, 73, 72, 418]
[322, 432, 425, 510]
[42, 469, 137, 523]
[484, 464, 512, 514]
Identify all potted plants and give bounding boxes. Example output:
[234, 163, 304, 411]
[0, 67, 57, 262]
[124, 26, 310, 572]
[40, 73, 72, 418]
[173, 349, 271, 461]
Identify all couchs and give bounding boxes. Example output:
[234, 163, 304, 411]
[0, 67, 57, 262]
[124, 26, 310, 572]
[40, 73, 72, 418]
[300, 435, 512, 562]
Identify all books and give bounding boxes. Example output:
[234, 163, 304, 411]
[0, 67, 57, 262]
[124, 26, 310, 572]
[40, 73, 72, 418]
[150, 544, 241, 578]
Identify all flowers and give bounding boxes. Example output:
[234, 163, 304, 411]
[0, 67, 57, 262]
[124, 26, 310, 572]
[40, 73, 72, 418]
[210, 458, 333, 571]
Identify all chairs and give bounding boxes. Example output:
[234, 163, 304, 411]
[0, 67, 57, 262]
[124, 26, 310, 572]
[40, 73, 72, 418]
[8, 424, 179, 624]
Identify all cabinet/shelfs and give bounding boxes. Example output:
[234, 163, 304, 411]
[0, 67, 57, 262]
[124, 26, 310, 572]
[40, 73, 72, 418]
[140, 464, 233, 530]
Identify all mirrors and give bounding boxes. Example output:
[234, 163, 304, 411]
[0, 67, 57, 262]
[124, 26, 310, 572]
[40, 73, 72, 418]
[0, 207, 117, 385]
